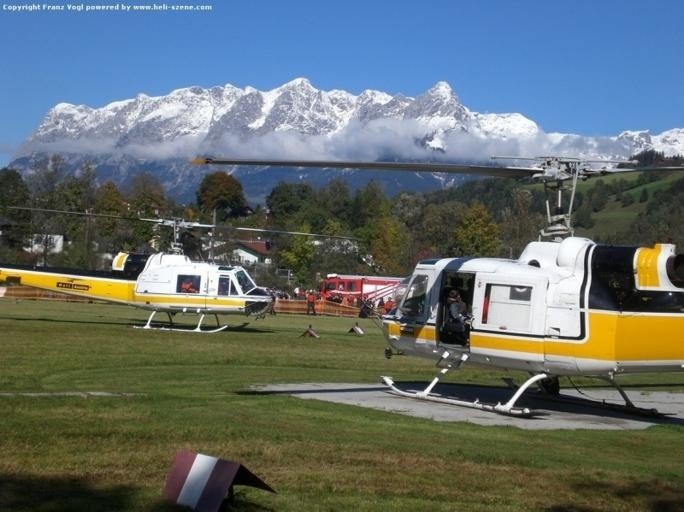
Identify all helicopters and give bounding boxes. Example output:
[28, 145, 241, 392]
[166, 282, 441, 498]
[188, 149, 684, 424]
[0, 203, 362, 334]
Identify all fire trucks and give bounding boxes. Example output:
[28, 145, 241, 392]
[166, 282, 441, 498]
[320, 271, 406, 314]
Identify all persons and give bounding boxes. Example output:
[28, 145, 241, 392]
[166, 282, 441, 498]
[444, 289, 470, 342]
[267, 283, 395, 315]
[181, 277, 198, 292]
[219, 279, 228, 295]
